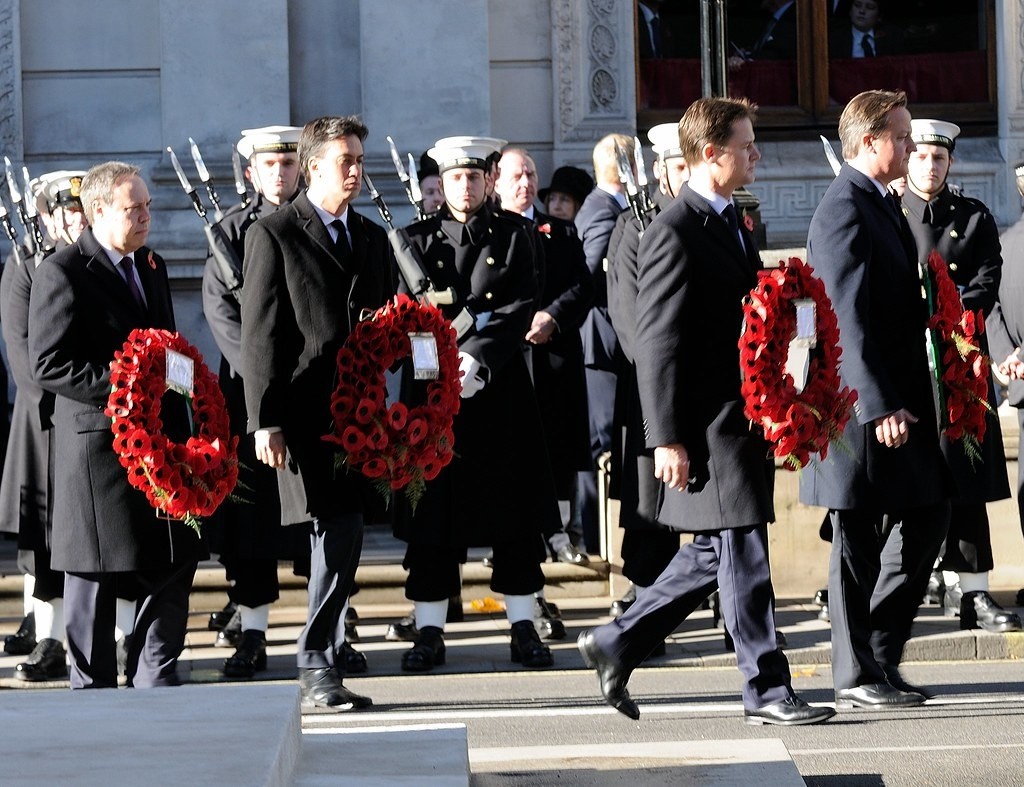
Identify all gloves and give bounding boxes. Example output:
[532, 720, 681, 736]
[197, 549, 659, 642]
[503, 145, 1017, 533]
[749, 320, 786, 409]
[455, 351, 486, 398]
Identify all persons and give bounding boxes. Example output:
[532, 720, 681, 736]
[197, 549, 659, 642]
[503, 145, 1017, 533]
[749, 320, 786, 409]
[5, 89, 1023, 731]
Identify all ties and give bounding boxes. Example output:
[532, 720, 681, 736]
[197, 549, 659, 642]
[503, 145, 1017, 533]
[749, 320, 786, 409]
[886, 189, 902, 226]
[118, 257, 147, 315]
[328, 220, 354, 275]
[723, 205, 740, 231]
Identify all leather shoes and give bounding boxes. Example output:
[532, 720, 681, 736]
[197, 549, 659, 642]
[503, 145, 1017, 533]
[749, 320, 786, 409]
[209, 600, 269, 680]
[834, 683, 927, 709]
[606, 574, 1024, 658]
[481, 553, 493, 567]
[3, 610, 131, 682]
[387, 590, 567, 668]
[552, 545, 589, 565]
[883, 668, 925, 697]
[299, 605, 368, 710]
[576, 628, 641, 720]
[745, 694, 838, 725]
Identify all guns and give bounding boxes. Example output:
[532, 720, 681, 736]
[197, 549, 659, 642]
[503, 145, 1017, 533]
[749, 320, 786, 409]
[613, 137, 636, 216]
[3, 155, 35, 254]
[0, 196, 24, 265]
[386, 136, 417, 208]
[634, 135, 649, 210]
[230, 144, 249, 209]
[21, 166, 44, 255]
[620, 148, 645, 239]
[406, 152, 426, 220]
[820, 134, 842, 177]
[186, 136, 226, 222]
[166, 145, 244, 308]
[360, 168, 477, 346]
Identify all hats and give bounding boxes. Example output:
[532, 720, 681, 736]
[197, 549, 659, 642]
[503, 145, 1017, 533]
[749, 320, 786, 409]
[237, 126, 305, 165]
[427, 135, 509, 176]
[647, 123, 685, 161]
[28, 168, 89, 215]
[910, 118, 962, 153]
[537, 166, 593, 211]
